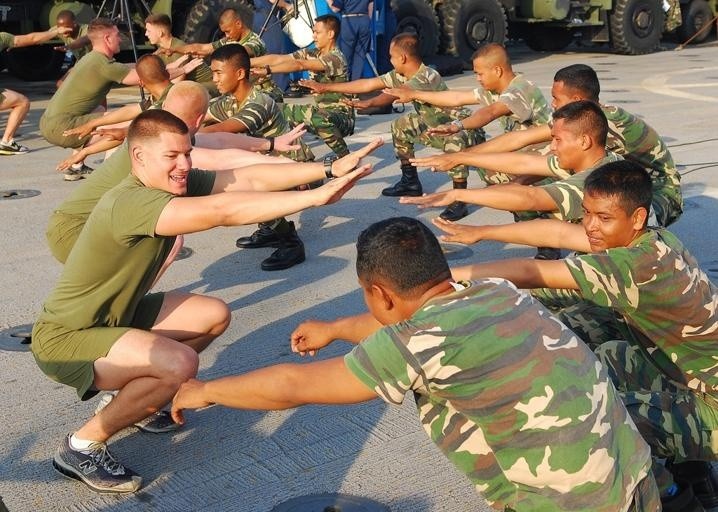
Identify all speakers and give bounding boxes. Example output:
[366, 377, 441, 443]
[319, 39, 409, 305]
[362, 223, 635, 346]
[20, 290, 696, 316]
[439, 180, 468, 221]
[260, 221, 305, 270]
[236, 225, 279, 248]
[535, 244, 561, 261]
[382, 165, 422, 196]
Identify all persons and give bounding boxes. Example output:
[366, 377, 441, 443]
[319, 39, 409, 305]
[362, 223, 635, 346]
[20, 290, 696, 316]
[38, 1, 356, 270]
[30, 106, 387, 495]
[32, 0, 717, 351]
[0, 22, 73, 155]
[165, 214, 668, 511]
[290, 32, 683, 261]
[429, 158, 718, 511]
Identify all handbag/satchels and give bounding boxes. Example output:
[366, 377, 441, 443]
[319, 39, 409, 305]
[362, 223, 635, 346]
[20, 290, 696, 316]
[323, 155, 336, 181]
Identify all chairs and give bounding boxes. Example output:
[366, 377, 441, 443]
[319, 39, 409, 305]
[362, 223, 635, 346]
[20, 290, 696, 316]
[63, 164, 93, 181]
[95, 392, 180, 432]
[51, 433, 142, 497]
[0, 138, 29, 155]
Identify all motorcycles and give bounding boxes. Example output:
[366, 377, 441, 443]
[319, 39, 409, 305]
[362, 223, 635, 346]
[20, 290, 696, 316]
[259, 0, 314, 38]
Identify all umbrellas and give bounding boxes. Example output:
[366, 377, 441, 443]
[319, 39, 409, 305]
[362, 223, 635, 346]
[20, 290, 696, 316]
[283, 87, 302, 98]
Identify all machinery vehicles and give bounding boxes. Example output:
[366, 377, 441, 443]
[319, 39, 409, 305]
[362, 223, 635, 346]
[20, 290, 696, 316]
[0, 0, 718, 83]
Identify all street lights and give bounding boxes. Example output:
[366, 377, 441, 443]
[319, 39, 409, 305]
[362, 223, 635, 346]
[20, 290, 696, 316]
[356, 90, 405, 115]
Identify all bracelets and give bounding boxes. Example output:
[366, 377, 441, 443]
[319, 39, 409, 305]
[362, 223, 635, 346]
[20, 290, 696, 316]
[323, 155, 336, 181]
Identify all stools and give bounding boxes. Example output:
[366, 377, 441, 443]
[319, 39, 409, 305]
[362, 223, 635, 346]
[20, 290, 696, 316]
[255, 8, 265, 12]
[342, 13, 364, 17]
